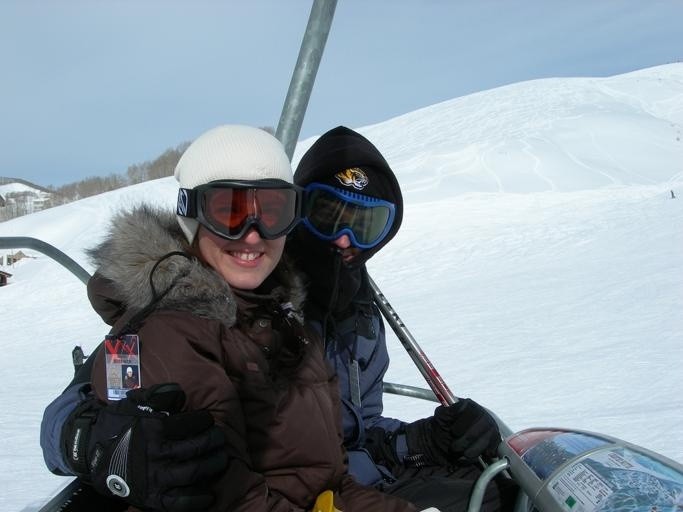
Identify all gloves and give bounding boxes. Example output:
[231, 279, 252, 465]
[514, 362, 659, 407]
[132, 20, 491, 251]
[392, 398, 502, 472]
[65, 383, 230, 512]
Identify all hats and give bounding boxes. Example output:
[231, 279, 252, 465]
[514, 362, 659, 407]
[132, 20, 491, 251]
[126, 366, 132, 373]
[174, 123, 296, 244]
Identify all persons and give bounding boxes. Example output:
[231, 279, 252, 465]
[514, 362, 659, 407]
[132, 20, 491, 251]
[87, 125, 416, 511]
[124, 366, 138, 389]
[38, 124, 501, 512]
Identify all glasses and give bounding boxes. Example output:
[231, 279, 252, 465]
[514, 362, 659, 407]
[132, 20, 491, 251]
[177, 178, 302, 240]
[302, 184, 397, 248]
